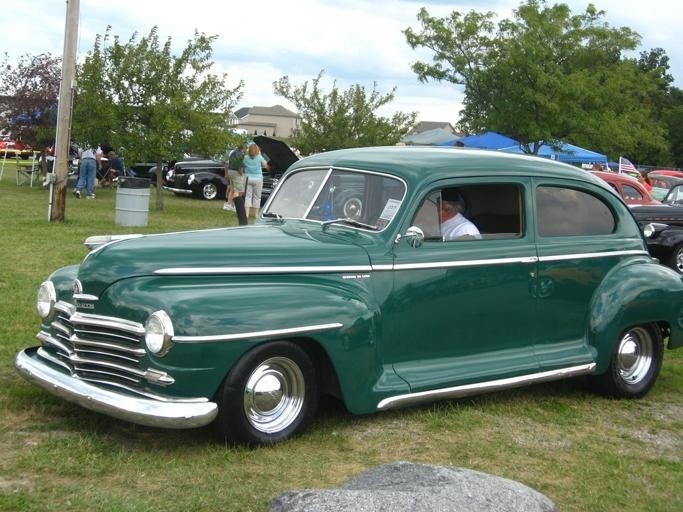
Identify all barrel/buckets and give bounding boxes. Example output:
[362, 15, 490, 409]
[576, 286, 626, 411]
[115, 175, 151, 227]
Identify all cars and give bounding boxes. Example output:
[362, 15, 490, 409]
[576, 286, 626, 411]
[161, 134, 299, 200]
[13, 145, 683, 444]
[586, 167, 683, 271]
[0, 134, 183, 177]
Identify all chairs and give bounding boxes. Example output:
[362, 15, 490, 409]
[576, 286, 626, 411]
[466, 207, 501, 234]
[15, 153, 40, 187]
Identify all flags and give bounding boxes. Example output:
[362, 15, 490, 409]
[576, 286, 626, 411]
[619, 157, 640, 174]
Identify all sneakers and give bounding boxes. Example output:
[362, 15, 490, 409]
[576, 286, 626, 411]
[222, 198, 237, 213]
[72, 188, 97, 200]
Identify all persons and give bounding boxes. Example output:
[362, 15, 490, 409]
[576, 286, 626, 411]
[415, 187, 483, 240]
[637, 170, 653, 192]
[37, 143, 124, 200]
[592, 163, 603, 172]
[220, 141, 270, 219]
